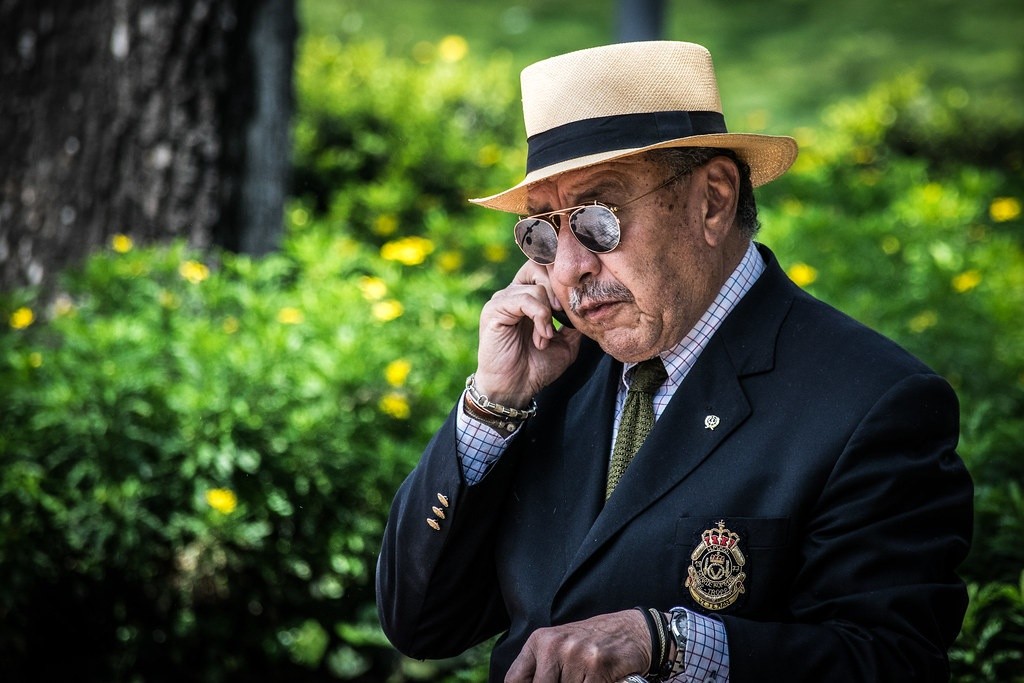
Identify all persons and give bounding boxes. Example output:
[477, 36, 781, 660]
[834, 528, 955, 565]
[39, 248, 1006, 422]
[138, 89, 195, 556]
[374, 41, 976, 683]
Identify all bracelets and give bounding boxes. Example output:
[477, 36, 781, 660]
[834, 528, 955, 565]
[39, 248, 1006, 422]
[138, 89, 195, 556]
[464, 373, 537, 432]
[634, 605, 671, 682]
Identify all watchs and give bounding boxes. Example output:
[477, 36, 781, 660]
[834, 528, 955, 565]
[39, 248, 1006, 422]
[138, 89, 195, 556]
[667, 610, 688, 679]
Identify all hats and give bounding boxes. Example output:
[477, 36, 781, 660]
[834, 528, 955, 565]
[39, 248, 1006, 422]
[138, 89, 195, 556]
[466, 38, 800, 217]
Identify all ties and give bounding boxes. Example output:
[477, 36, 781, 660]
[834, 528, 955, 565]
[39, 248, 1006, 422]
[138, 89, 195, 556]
[606, 357, 671, 501]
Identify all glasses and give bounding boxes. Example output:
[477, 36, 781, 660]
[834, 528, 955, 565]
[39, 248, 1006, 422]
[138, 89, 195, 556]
[514, 160, 710, 266]
